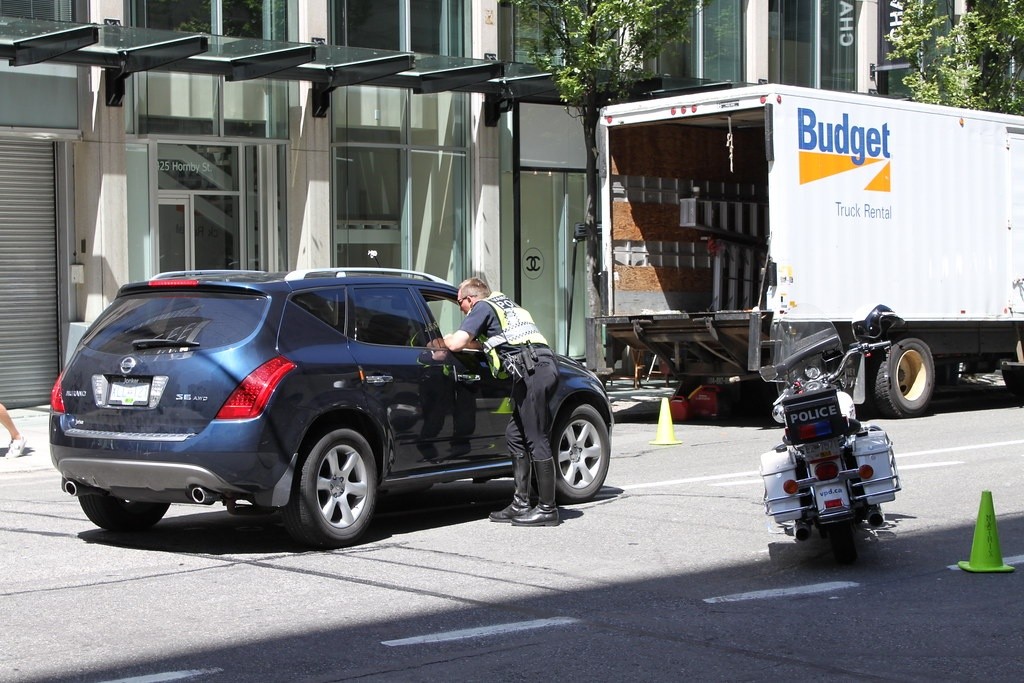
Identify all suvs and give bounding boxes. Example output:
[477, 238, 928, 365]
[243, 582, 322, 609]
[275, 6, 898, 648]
[49, 265, 615, 552]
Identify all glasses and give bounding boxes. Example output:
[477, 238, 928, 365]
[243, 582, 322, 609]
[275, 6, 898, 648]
[456, 295, 477, 306]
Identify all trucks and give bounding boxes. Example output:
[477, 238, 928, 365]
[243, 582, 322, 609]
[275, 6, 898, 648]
[593, 82, 1024, 417]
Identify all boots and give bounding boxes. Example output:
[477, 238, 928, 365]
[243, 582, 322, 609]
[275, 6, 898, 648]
[488, 454, 532, 523]
[451, 415, 476, 456]
[417, 415, 445, 458]
[512, 456, 559, 526]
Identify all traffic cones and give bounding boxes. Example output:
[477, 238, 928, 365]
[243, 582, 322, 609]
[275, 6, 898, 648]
[648, 397, 684, 445]
[957, 491, 1017, 574]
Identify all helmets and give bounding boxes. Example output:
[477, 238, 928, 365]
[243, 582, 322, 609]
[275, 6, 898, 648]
[852, 304, 905, 343]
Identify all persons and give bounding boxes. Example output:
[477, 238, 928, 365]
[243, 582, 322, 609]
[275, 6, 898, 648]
[415, 338, 477, 459]
[443, 276, 560, 527]
[0, 404, 27, 459]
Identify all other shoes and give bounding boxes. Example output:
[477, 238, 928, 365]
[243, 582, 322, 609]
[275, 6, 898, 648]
[5, 435, 27, 459]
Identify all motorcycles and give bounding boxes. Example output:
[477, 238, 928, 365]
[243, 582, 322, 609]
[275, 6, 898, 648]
[757, 304, 901, 565]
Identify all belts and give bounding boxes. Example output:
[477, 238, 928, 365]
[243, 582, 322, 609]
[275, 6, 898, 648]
[517, 348, 554, 361]
